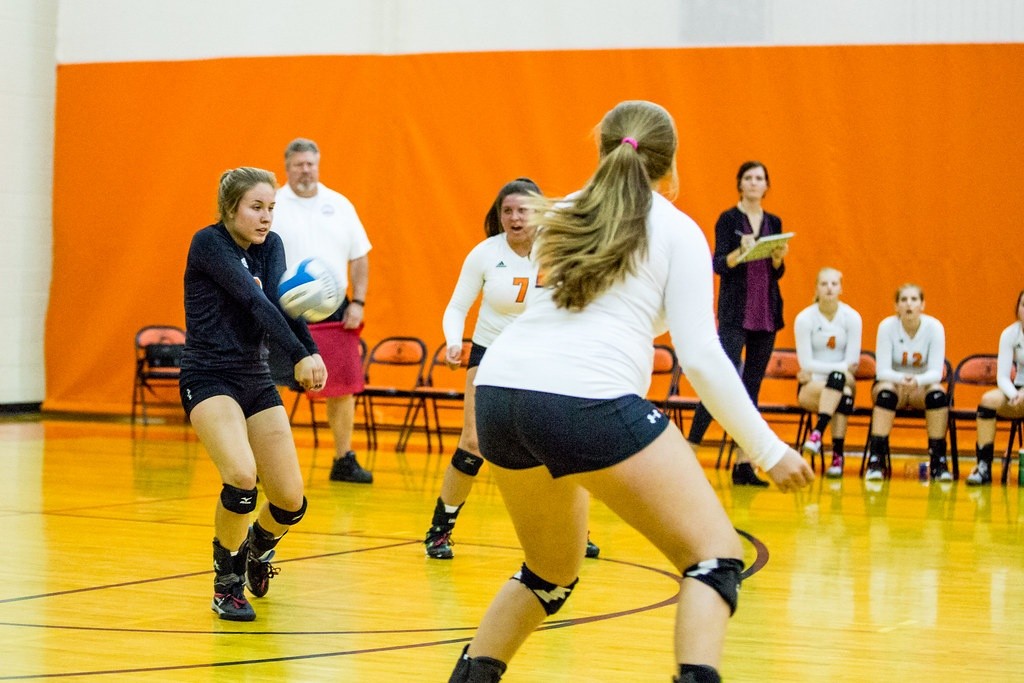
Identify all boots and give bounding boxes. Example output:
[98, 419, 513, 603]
[238, 518, 289, 598]
[212, 538, 257, 623]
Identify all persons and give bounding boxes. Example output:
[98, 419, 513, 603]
[865, 284, 952, 482]
[688, 161, 785, 487]
[179, 166, 328, 622]
[424, 177, 600, 559]
[269, 138, 373, 484]
[966, 291, 1024, 485]
[794, 267, 862, 478]
[449, 99, 816, 683]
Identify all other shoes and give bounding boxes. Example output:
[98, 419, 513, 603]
[731, 463, 770, 486]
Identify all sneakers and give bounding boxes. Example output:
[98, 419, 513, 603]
[803, 429, 823, 454]
[586, 530, 601, 558]
[865, 452, 885, 480]
[828, 451, 845, 476]
[424, 525, 455, 560]
[965, 458, 993, 484]
[929, 455, 953, 481]
[329, 450, 374, 483]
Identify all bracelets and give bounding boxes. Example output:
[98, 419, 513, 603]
[352, 299, 365, 307]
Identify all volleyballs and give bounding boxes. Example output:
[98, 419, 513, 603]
[278, 256, 347, 323]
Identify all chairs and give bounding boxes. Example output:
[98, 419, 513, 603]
[290, 335, 1024, 487]
[131, 324, 187, 425]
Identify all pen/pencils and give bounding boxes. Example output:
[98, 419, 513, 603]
[735, 230, 743, 236]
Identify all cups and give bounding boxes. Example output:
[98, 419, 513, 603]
[1019, 449, 1024, 486]
